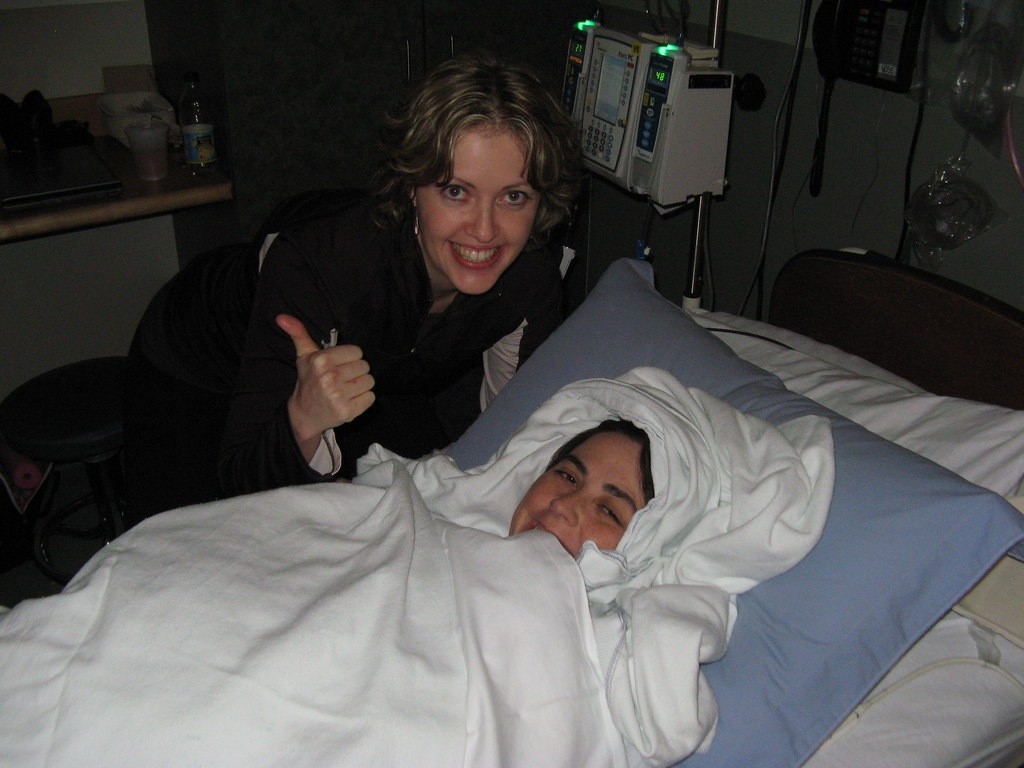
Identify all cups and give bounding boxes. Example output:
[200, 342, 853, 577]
[124, 120, 170, 182]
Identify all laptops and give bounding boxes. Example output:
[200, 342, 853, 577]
[0, 143, 123, 212]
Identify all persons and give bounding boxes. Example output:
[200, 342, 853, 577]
[118, 55, 595, 535]
[507, 420, 653, 560]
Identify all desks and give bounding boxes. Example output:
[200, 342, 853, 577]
[0, 91, 236, 245]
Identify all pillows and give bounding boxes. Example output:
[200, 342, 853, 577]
[452, 252, 1024, 768]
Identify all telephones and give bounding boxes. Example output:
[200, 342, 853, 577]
[811, 0, 929, 93]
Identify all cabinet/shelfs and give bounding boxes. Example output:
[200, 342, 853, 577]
[144, 0, 604, 276]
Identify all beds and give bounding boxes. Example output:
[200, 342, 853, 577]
[0, 245, 1024, 768]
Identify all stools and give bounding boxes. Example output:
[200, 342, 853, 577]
[0, 356, 146, 585]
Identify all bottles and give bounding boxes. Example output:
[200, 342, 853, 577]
[178, 70, 218, 176]
